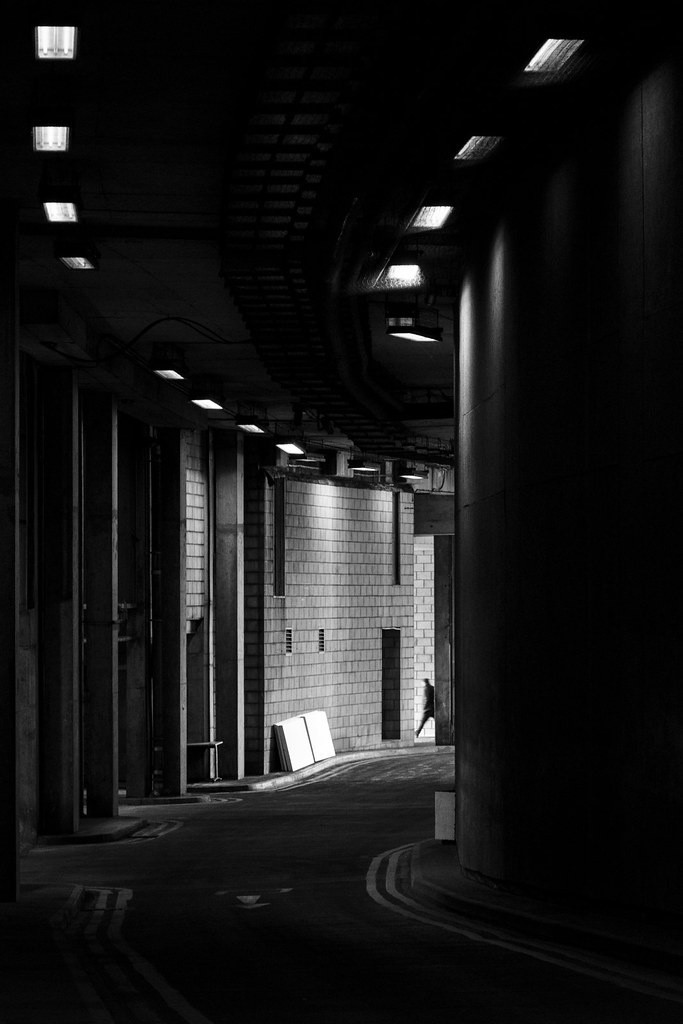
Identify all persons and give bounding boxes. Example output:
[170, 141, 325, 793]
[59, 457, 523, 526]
[415, 679, 434, 737]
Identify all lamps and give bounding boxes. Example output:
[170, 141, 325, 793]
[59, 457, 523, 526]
[384, 302, 443, 343]
[236, 402, 268, 434]
[289, 440, 326, 462]
[191, 379, 224, 411]
[274, 424, 304, 455]
[151, 343, 186, 380]
[399, 467, 430, 481]
[348, 447, 380, 471]
